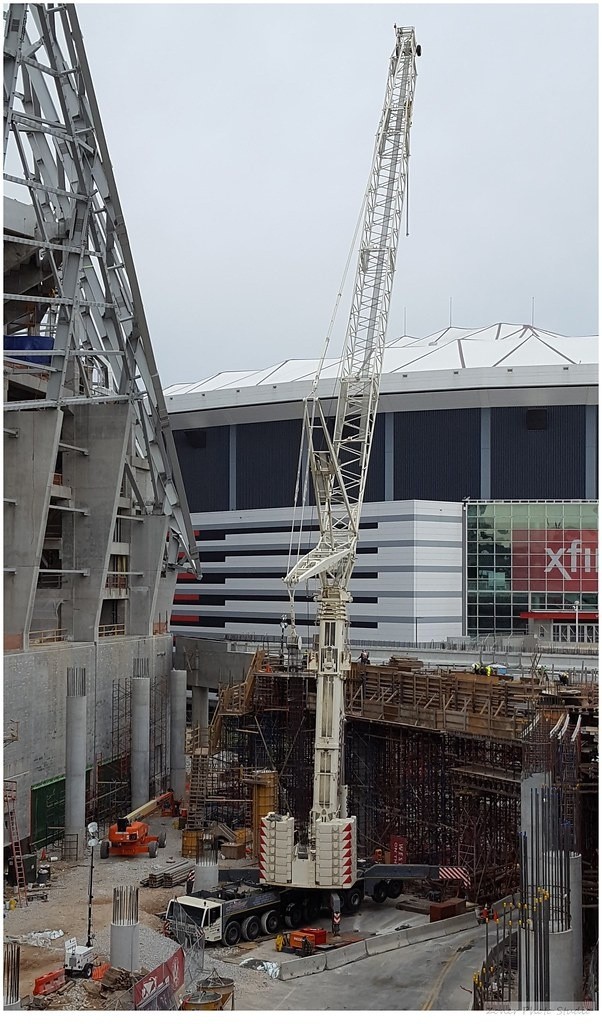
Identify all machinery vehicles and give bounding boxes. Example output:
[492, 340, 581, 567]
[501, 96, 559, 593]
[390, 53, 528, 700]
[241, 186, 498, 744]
[100, 792, 176, 859]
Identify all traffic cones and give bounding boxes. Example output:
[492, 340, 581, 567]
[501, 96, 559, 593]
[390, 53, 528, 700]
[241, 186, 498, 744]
[40, 847, 48, 861]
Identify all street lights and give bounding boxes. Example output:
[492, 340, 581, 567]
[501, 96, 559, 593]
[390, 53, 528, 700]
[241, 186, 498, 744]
[473, 887, 550, 1011]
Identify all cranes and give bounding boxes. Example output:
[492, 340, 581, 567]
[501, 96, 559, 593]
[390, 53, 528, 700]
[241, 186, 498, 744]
[163, 23, 472, 948]
